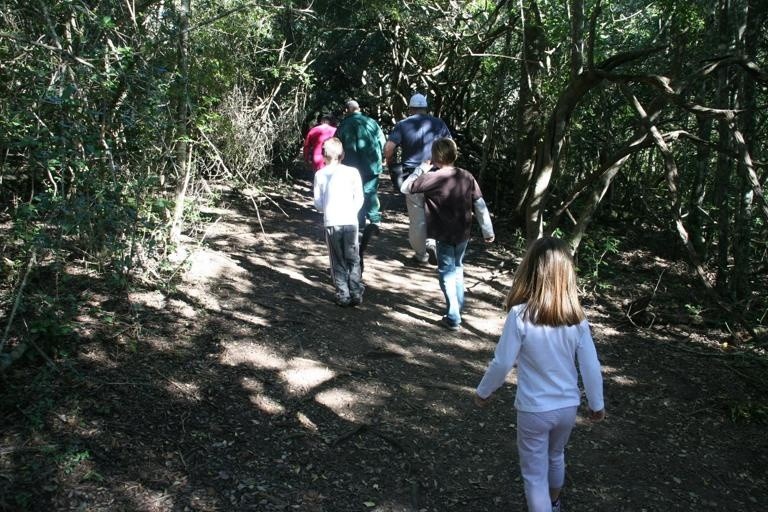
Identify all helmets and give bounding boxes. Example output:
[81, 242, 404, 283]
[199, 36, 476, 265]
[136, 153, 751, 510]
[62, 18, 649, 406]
[408, 94, 427, 109]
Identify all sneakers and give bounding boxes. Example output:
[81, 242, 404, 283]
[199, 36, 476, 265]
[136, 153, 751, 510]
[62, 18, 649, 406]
[425, 245, 437, 265]
[442, 315, 459, 330]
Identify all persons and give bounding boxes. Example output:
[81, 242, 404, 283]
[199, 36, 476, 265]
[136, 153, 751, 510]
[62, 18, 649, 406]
[384, 93, 453, 266]
[314, 137, 365, 307]
[401, 137, 495, 330]
[475, 237, 606, 512]
[333, 100, 388, 234]
[304, 112, 338, 171]
